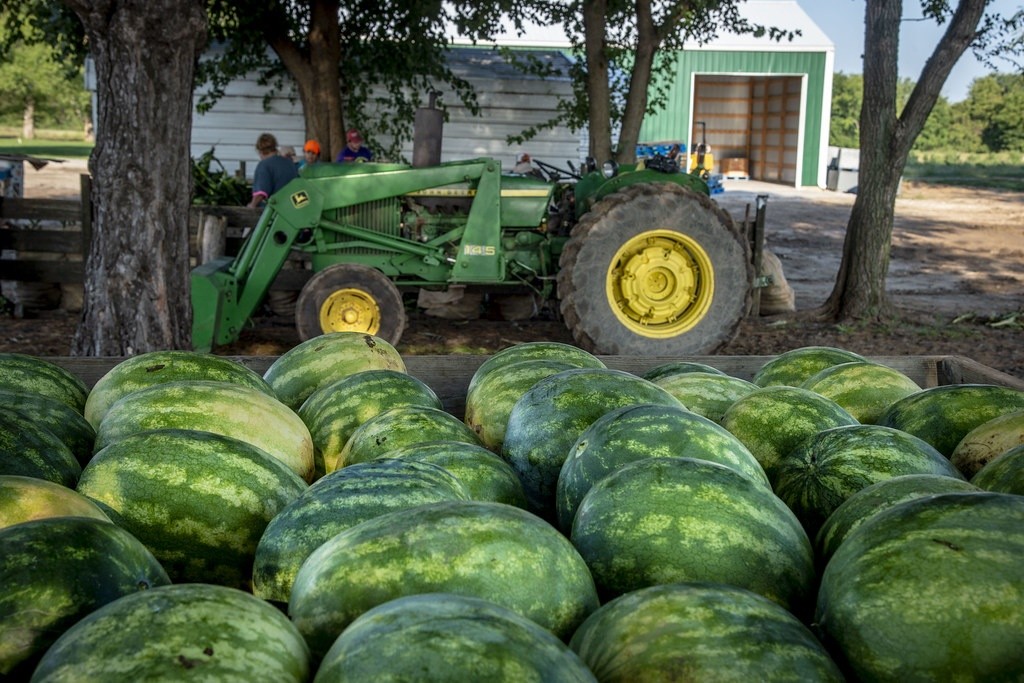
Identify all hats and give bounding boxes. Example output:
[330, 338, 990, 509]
[346, 129, 364, 143]
[303, 140, 320, 154]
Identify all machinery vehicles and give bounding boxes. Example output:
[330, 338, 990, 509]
[188, 93, 775, 354]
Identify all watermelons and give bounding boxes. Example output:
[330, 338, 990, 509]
[0, 332, 1024, 683]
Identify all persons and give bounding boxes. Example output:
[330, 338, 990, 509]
[242, 133, 324, 238]
[511, 154, 535, 177]
[337, 129, 371, 162]
